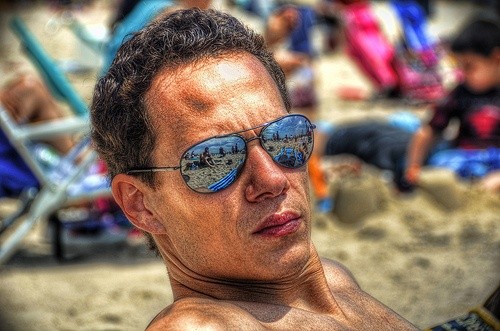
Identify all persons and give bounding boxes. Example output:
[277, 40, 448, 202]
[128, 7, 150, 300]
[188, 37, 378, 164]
[90, 11, 415, 329]
[185, 125, 310, 169]
[402, 17, 500, 202]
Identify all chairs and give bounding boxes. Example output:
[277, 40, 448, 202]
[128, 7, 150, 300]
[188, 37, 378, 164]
[0, 15, 159, 267]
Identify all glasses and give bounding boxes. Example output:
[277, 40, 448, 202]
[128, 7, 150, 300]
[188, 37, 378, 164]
[122, 114, 317, 195]
